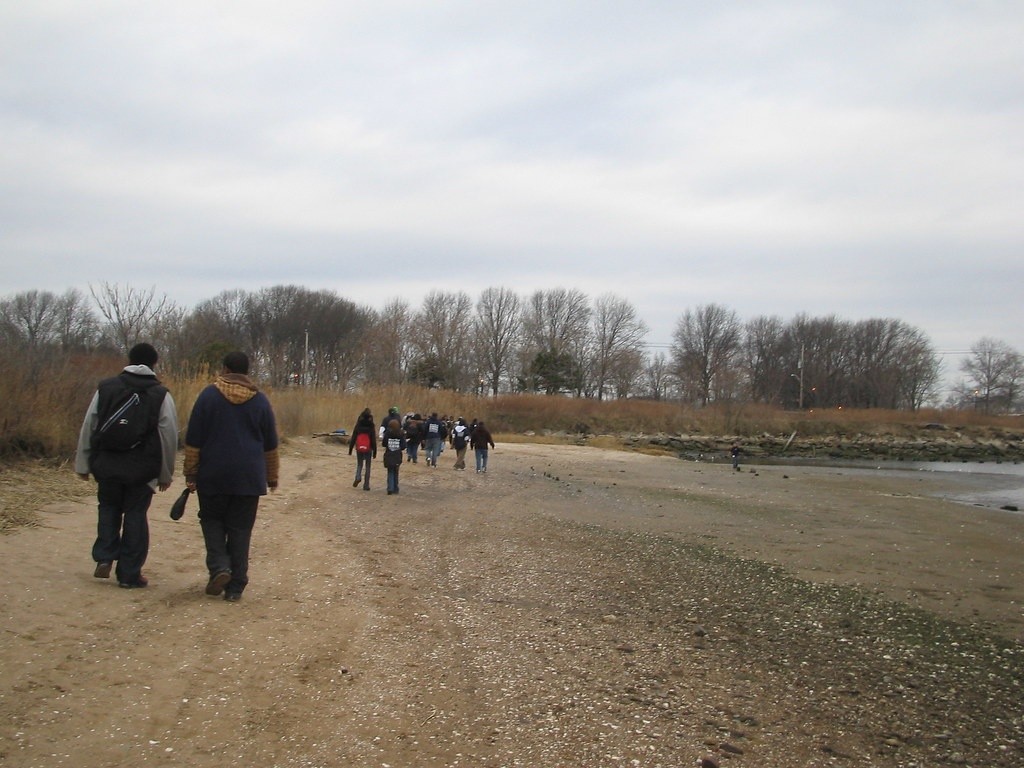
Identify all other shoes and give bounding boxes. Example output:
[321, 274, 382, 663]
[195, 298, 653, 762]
[407, 456, 411, 462]
[427, 458, 436, 469]
[477, 470, 481, 473]
[482, 466, 487, 472]
[205, 566, 232, 596]
[364, 487, 370, 491]
[225, 591, 241, 601]
[413, 461, 417, 464]
[388, 486, 399, 495]
[120, 570, 148, 589]
[94, 559, 112, 578]
[353, 479, 361, 487]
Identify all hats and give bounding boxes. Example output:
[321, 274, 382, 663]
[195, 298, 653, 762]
[391, 407, 399, 413]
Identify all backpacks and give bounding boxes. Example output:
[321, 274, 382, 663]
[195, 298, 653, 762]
[387, 430, 404, 452]
[406, 427, 419, 445]
[438, 425, 448, 439]
[356, 425, 373, 453]
[91, 374, 161, 453]
[453, 428, 468, 450]
[428, 419, 440, 434]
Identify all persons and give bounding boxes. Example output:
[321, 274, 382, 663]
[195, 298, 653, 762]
[347, 408, 377, 490]
[378, 406, 494, 474]
[75, 343, 178, 587]
[182, 350, 279, 601]
[382, 420, 406, 494]
